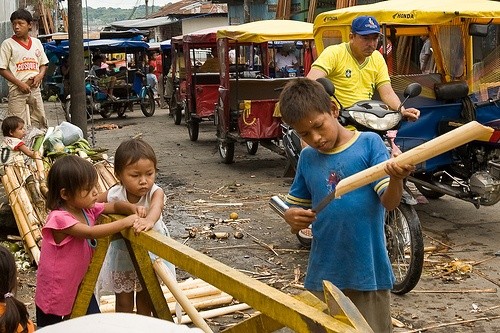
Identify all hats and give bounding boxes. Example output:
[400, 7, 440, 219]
[351, 16, 384, 36]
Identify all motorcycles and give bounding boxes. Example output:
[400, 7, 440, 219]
[39, 0, 499, 207]
[268, 77, 424, 298]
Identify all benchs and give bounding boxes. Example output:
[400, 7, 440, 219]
[389, 72, 443, 106]
[194, 75, 220, 85]
[230, 78, 295, 113]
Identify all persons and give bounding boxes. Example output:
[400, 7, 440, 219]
[419, 34, 439, 74]
[146, 65, 158, 98]
[302, 40, 313, 77]
[0, 245, 35, 333]
[35, 156, 147, 330]
[228, 47, 241, 64]
[0, 8, 49, 132]
[0, 116, 43, 178]
[94, 139, 175, 316]
[254, 49, 260, 64]
[195, 47, 221, 73]
[279, 79, 416, 333]
[274, 43, 298, 72]
[88, 54, 127, 101]
[305, 16, 420, 125]
[47, 55, 71, 101]
[167, 48, 185, 78]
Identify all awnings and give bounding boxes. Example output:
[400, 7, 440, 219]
[110, 17, 179, 28]
